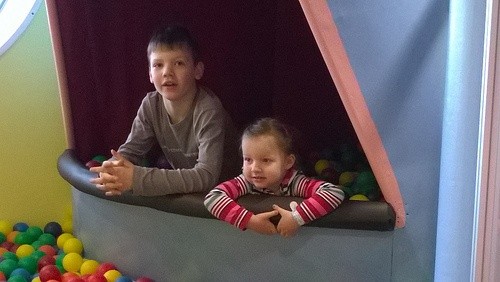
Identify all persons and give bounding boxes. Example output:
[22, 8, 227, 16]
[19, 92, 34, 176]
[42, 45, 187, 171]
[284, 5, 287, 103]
[202, 116, 345, 239]
[89, 25, 226, 197]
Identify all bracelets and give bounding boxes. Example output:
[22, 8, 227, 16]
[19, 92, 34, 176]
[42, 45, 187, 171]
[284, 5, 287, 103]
[289, 200, 305, 228]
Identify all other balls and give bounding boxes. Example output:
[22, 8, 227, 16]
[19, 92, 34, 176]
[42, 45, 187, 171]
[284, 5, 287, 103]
[307, 144, 385, 200]
[84, 145, 170, 180]
[0, 215, 157, 282]
